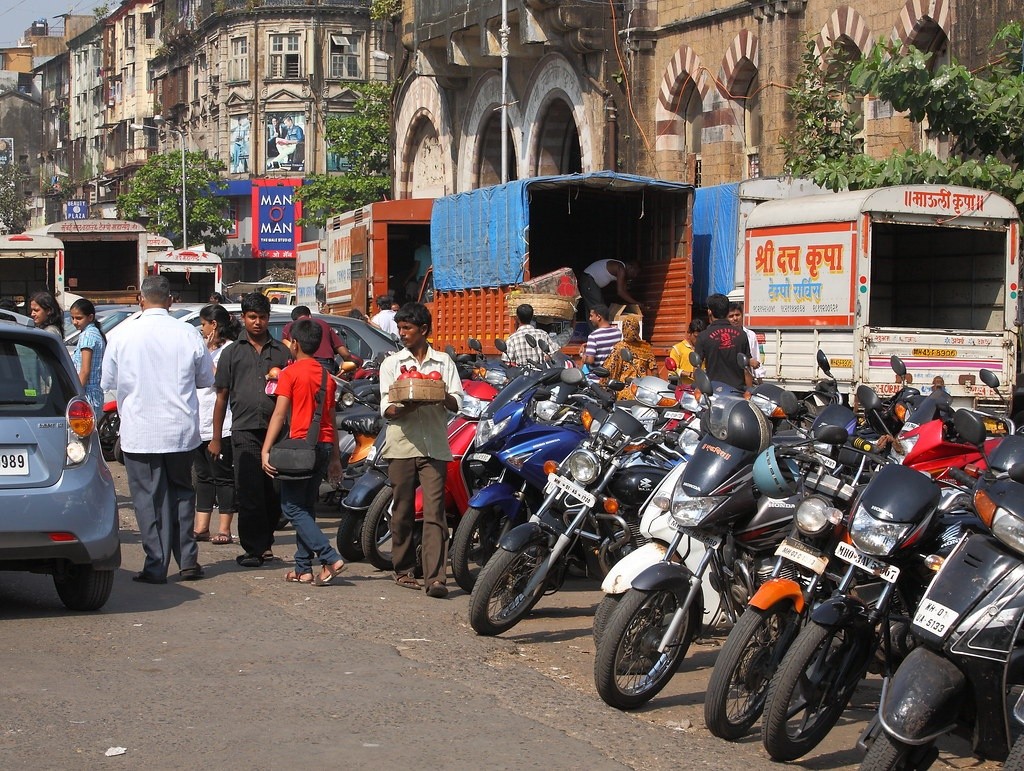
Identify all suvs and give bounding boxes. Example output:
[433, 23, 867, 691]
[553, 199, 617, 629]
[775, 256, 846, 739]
[0, 307, 121, 612]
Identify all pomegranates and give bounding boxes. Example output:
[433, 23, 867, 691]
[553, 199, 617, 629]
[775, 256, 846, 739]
[395, 364, 442, 380]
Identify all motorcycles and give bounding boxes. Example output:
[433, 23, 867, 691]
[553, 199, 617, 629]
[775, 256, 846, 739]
[604, 349, 1024, 770]
[333, 335, 745, 637]
[98, 401, 124, 465]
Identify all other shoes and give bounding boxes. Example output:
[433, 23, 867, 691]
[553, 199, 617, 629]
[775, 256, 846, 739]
[236, 554, 264, 567]
[133, 571, 168, 584]
[180, 569, 205, 577]
[263, 550, 274, 561]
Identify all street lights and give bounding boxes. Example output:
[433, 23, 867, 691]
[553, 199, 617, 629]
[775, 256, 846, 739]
[129, 113, 188, 252]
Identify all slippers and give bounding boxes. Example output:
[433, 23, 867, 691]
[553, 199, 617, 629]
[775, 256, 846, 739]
[287, 568, 314, 583]
[194, 529, 210, 542]
[211, 533, 233, 545]
[312, 557, 348, 585]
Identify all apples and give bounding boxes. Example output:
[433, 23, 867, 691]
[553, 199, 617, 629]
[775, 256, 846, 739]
[268, 366, 281, 377]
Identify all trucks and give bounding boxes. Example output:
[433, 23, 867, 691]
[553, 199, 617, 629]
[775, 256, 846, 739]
[694, 172, 1020, 415]
[0, 235, 65, 320]
[21, 219, 148, 315]
[295, 169, 691, 363]
[262, 288, 296, 306]
[147, 232, 223, 303]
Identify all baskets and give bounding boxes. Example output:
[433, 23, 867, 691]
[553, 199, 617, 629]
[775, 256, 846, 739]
[507, 293, 580, 323]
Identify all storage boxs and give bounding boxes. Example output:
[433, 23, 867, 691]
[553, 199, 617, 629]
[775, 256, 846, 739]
[520, 266, 581, 299]
[608, 302, 643, 342]
[550, 321, 591, 342]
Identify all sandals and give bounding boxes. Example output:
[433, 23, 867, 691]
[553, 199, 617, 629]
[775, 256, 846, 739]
[426, 580, 448, 598]
[392, 572, 421, 590]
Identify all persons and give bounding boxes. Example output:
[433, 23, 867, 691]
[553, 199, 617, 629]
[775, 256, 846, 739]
[282, 305, 349, 374]
[348, 295, 400, 340]
[260, 319, 347, 587]
[239, 293, 248, 302]
[191, 304, 240, 544]
[379, 303, 465, 598]
[69, 299, 106, 423]
[580, 258, 646, 330]
[209, 292, 222, 305]
[100, 274, 213, 585]
[29, 291, 64, 345]
[401, 232, 434, 300]
[206, 291, 293, 567]
[669, 294, 765, 399]
[502, 304, 560, 367]
[580, 305, 622, 369]
[600, 318, 660, 401]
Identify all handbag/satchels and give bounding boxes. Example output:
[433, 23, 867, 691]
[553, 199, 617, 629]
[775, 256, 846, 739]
[269, 439, 320, 480]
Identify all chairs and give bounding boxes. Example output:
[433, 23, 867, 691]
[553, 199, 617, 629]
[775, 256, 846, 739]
[0, 377, 28, 400]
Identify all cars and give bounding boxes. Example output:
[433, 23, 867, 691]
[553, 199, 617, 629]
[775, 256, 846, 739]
[46, 303, 403, 400]
